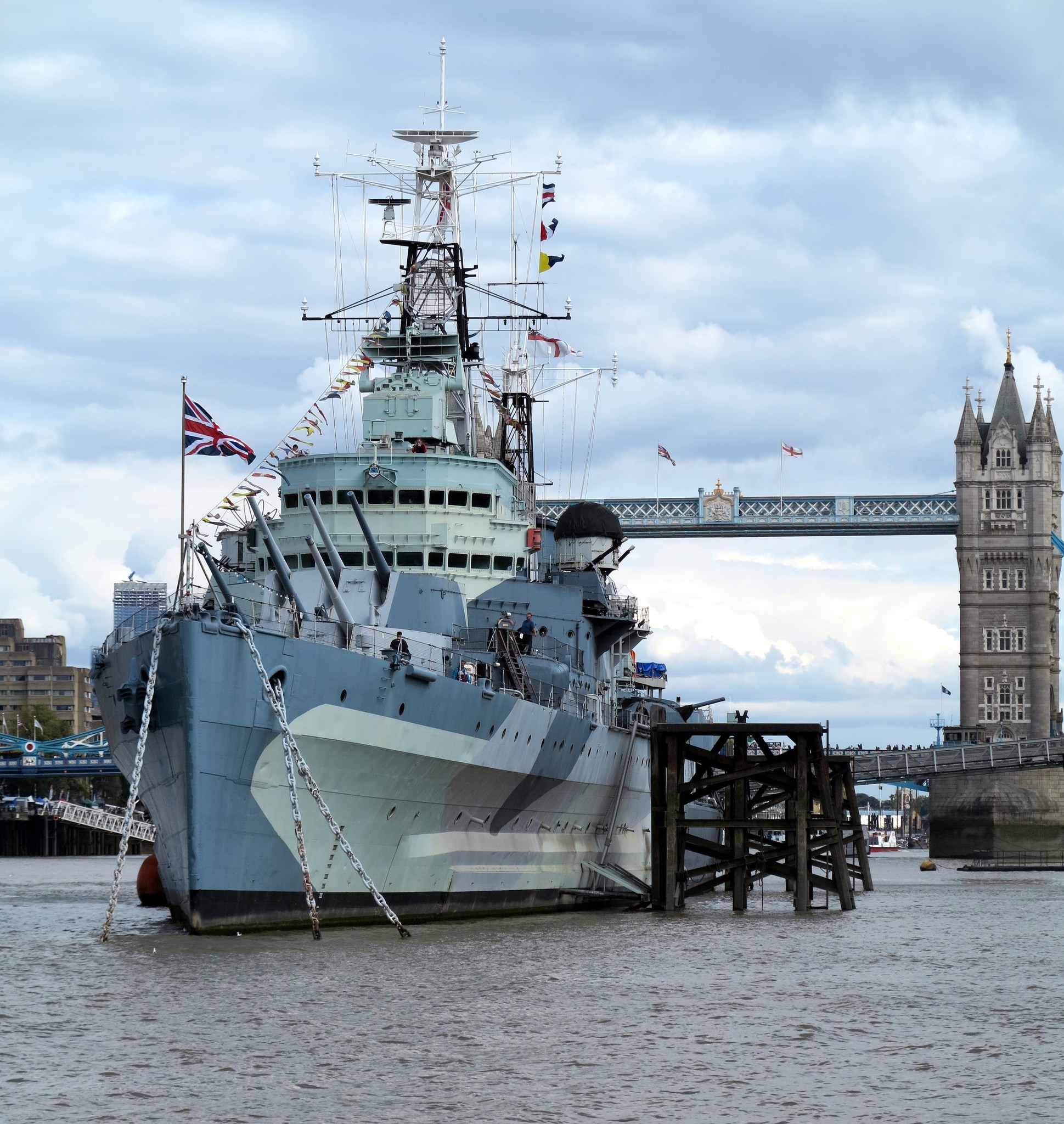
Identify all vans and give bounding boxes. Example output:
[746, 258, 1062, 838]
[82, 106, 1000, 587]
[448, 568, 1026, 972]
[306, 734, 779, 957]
[748, 739, 785, 749]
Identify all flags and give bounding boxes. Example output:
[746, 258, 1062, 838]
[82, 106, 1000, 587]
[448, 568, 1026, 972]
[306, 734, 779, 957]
[194, 480, 269, 547]
[295, 403, 328, 437]
[2, 716, 43, 737]
[319, 347, 372, 401]
[183, 394, 256, 465]
[390, 257, 429, 315]
[782, 442, 803, 459]
[539, 183, 564, 272]
[942, 686, 951, 696]
[252, 435, 315, 486]
[528, 328, 583, 358]
[481, 371, 522, 430]
[658, 446, 676, 466]
[362, 311, 391, 349]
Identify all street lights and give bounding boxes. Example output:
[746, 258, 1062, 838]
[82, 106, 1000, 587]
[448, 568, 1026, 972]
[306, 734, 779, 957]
[999, 718, 1003, 742]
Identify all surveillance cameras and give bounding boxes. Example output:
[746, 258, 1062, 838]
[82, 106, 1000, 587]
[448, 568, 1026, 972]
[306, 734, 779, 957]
[466, 432, 470, 436]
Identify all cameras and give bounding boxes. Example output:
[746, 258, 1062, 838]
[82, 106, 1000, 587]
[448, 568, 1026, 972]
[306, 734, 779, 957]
[417, 443, 421, 447]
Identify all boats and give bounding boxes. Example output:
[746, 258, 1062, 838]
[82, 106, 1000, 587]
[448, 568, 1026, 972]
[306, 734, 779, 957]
[869, 830, 899, 853]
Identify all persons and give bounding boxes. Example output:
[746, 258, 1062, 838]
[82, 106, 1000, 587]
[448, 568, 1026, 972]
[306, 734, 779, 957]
[452, 660, 471, 683]
[520, 613, 537, 656]
[763, 834, 772, 845]
[634, 702, 650, 725]
[390, 631, 409, 652]
[412, 438, 427, 453]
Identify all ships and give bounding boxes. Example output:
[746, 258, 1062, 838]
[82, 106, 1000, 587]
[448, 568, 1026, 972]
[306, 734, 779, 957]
[87, 32, 729, 937]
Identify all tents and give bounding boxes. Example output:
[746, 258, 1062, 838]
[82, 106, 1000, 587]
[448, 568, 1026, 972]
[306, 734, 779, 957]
[34, 797, 105, 808]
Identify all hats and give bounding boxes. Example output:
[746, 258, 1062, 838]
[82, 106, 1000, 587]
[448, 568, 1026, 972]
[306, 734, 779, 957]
[397, 632, 402, 636]
